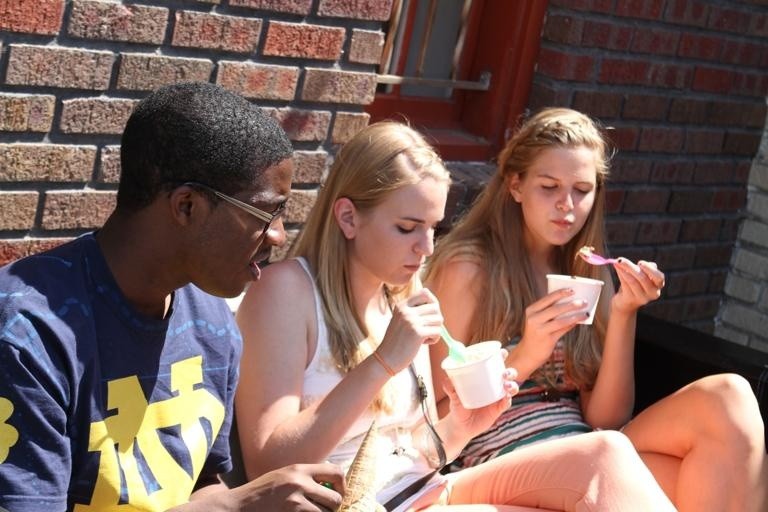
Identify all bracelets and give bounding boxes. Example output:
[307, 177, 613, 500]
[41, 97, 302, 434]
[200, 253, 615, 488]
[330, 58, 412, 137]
[373, 350, 398, 379]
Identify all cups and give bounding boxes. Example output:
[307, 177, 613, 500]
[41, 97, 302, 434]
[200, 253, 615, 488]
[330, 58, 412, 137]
[439, 339, 507, 412]
[543, 273, 604, 327]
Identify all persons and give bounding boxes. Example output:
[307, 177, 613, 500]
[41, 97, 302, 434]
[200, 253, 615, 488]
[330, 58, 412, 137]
[0, 81, 346, 511]
[233, 117, 678, 512]
[417, 106, 768, 511]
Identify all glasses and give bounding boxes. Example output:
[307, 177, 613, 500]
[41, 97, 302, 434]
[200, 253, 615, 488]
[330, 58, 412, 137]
[185, 182, 285, 233]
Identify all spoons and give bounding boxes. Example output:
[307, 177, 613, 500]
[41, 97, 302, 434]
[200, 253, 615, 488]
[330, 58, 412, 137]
[579, 251, 620, 268]
[438, 326, 468, 363]
